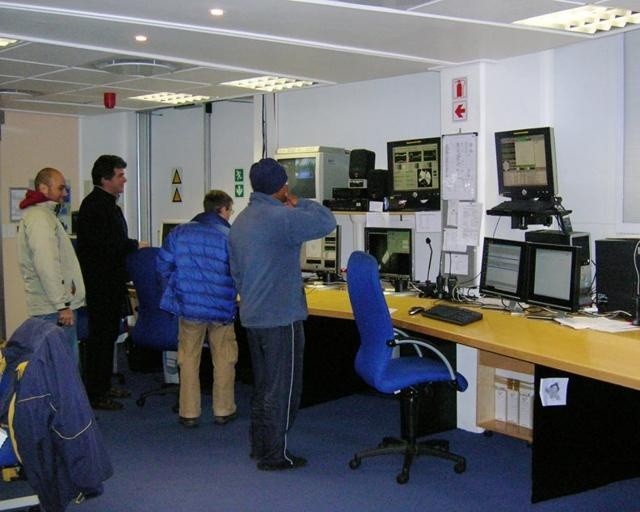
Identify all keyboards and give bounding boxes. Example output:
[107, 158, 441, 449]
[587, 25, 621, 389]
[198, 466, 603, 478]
[422, 303, 483, 327]
[492, 201, 547, 213]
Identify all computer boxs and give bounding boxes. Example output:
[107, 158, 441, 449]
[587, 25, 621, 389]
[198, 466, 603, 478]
[525, 229, 593, 307]
[595, 237, 640, 325]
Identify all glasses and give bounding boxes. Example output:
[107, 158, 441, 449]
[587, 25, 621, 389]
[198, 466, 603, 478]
[227, 208, 235, 215]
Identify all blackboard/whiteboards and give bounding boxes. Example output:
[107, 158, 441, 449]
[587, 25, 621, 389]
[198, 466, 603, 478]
[441, 132, 478, 202]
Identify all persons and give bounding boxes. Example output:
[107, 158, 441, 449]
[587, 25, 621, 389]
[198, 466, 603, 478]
[226, 157, 337, 471]
[17, 167, 88, 366]
[74, 154, 151, 410]
[153, 190, 239, 429]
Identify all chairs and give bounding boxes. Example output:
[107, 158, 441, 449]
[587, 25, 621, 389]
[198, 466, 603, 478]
[0, 318, 64, 510]
[346, 249, 469, 484]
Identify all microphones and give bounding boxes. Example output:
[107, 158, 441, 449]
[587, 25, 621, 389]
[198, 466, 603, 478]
[418, 237, 436, 291]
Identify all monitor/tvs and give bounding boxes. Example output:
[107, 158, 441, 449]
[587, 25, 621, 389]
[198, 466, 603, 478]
[299, 224, 341, 287]
[387, 136, 440, 197]
[275, 145, 350, 204]
[364, 226, 416, 296]
[159, 219, 190, 247]
[495, 126, 558, 200]
[524, 242, 582, 314]
[479, 236, 525, 315]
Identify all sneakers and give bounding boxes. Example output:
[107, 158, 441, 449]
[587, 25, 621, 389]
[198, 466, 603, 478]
[257, 456, 307, 471]
[106, 387, 131, 398]
[98, 398, 123, 410]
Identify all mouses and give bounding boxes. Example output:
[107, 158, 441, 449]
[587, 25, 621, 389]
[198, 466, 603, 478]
[408, 306, 424, 315]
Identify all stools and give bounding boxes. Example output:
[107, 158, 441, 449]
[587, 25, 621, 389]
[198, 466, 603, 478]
[135, 344, 180, 414]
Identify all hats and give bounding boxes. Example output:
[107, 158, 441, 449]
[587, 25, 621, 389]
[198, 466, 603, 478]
[250, 158, 288, 194]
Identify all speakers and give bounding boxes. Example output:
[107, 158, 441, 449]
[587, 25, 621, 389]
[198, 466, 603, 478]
[348, 149, 375, 179]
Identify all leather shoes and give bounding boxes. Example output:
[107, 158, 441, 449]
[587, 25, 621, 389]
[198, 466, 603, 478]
[176, 412, 200, 429]
[215, 407, 240, 425]
